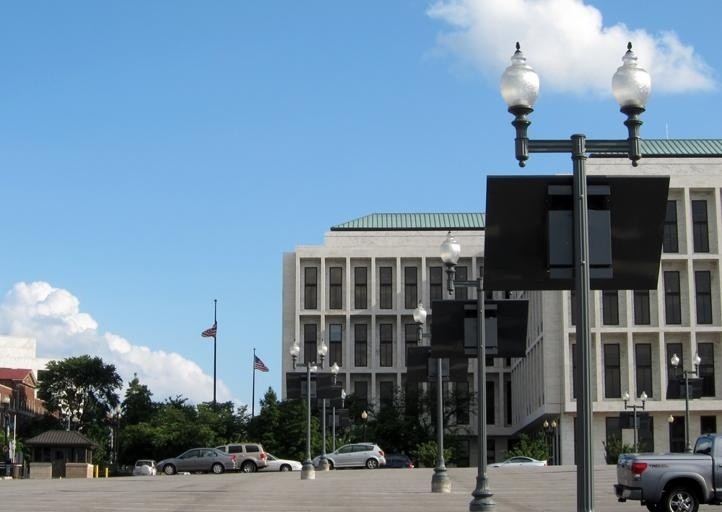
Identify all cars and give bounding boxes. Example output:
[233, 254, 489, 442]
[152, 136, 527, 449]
[155, 447, 238, 474]
[257, 453, 303, 472]
[311, 443, 387, 470]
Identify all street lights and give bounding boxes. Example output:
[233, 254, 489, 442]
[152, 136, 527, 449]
[362, 411, 367, 443]
[288, 338, 347, 480]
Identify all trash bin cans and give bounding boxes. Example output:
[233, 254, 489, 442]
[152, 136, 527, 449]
[12, 464, 23, 480]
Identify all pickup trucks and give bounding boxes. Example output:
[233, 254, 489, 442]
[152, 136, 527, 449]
[613, 433, 722, 512]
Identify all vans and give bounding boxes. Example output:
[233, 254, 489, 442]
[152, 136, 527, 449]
[204, 443, 269, 473]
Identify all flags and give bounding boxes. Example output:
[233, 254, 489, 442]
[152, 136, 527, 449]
[201, 323, 216, 336]
[254, 355, 269, 372]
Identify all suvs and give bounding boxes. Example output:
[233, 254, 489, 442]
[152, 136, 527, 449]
[133, 460, 158, 476]
